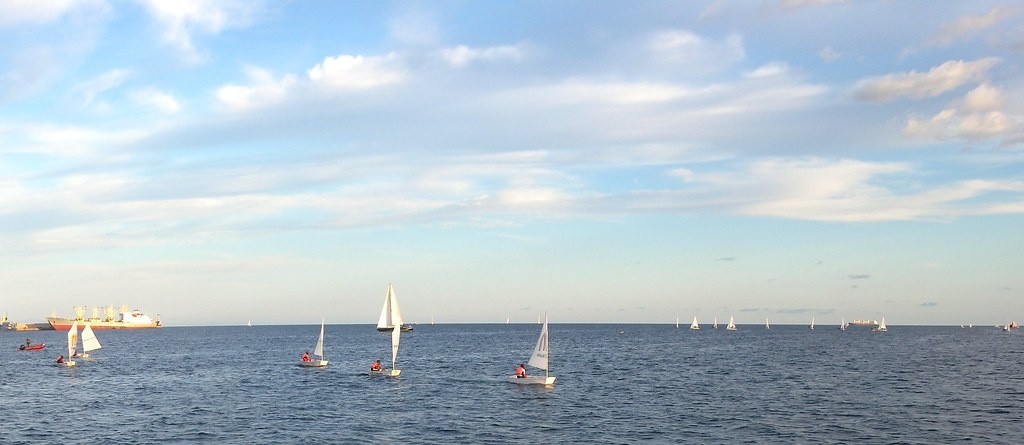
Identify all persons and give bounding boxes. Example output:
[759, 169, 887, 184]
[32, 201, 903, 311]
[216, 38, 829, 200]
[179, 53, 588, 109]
[300, 351, 312, 362]
[25, 337, 31, 347]
[56, 354, 64, 363]
[370, 360, 382, 372]
[515, 364, 527, 378]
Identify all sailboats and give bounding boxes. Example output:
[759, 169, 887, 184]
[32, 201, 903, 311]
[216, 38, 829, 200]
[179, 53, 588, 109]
[375, 285, 416, 332]
[958, 321, 1022, 333]
[370, 322, 402, 379]
[53, 322, 81, 368]
[504, 313, 557, 387]
[73, 324, 101, 359]
[296, 319, 327, 367]
[673, 313, 890, 335]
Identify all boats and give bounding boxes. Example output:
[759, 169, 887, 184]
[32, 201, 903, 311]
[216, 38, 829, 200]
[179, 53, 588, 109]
[17, 343, 45, 351]
[45, 303, 163, 335]
